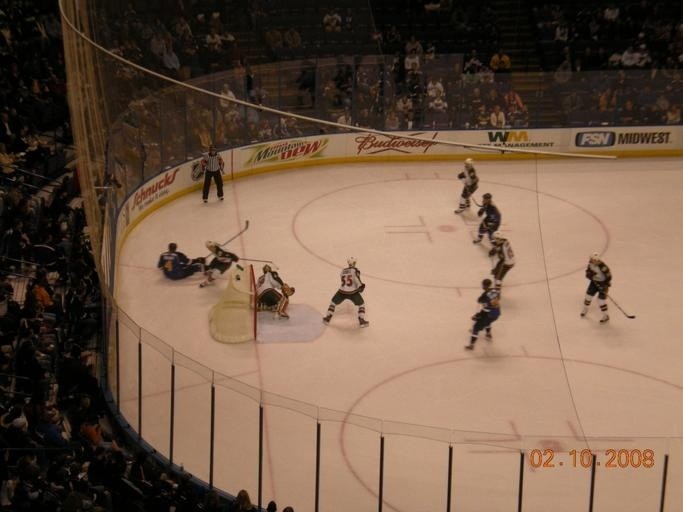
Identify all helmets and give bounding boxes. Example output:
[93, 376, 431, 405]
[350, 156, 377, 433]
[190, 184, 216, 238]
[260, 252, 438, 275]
[348, 256, 357, 265]
[464, 158, 503, 287]
[204, 240, 215, 247]
[590, 252, 600, 260]
[263, 264, 272, 272]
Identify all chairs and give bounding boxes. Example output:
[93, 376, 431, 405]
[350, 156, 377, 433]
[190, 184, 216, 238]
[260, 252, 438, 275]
[0, 0, 683, 512]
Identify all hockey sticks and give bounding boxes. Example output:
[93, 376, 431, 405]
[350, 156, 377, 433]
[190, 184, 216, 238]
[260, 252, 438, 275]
[594, 281, 636, 318]
[204, 220, 249, 258]
[239, 259, 279, 270]
[462, 178, 484, 207]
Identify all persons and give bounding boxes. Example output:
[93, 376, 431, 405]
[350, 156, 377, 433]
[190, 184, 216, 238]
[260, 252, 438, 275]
[471, 192, 501, 248]
[199, 238, 240, 289]
[201, 485, 294, 512]
[200, 144, 225, 203]
[256, 265, 293, 319]
[321, 255, 369, 326]
[463, 278, 500, 350]
[578, 250, 613, 324]
[199, 0, 682, 160]
[451, 158, 479, 215]
[2, 0, 206, 511]
[486, 231, 515, 300]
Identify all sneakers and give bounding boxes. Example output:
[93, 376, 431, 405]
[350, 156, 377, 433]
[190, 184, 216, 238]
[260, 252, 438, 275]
[581, 310, 610, 324]
[272, 309, 370, 326]
[465, 335, 492, 350]
